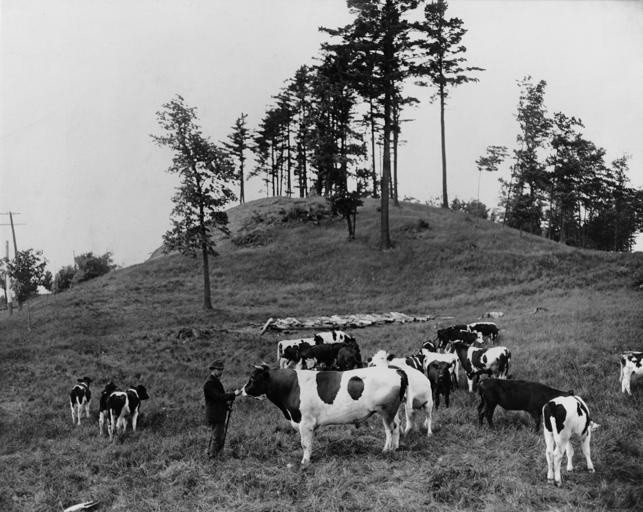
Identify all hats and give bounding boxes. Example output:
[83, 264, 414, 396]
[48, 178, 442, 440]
[209, 360, 224, 370]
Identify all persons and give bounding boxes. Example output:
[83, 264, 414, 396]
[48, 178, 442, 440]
[203, 358, 242, 460]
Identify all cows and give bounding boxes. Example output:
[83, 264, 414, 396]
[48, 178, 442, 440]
[366, 319, 514, 437]
[474, 377, 575, 434]
[276, 331, 364, 372]
[542, 393, 602, 489]
[98, 379, 150, 442]
[69, 375, 95, 427]
[618, 349, 643, 396]
[237, 360, 408, 468]
